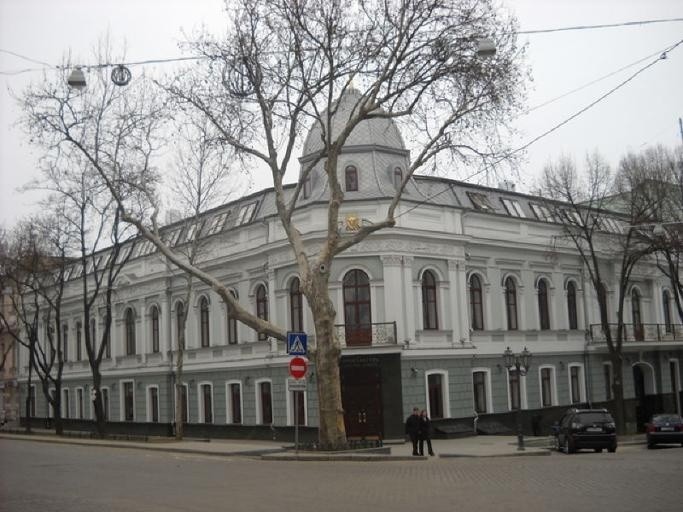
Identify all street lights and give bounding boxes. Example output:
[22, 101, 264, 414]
[500, 345, 535, 451]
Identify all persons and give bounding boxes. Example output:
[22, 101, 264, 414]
[418, 409, 437, 457]
[404, 407, 422, 455]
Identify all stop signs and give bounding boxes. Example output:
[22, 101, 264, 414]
[289, 356, 308, 381]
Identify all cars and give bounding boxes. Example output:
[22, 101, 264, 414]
[643, 413, 683, 449]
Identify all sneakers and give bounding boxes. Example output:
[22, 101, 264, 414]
[413, 452, 435, 456]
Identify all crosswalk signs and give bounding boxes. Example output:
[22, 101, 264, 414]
[287, 331, 308, 356]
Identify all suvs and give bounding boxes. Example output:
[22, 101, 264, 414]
[551, 406, 618, 454]
